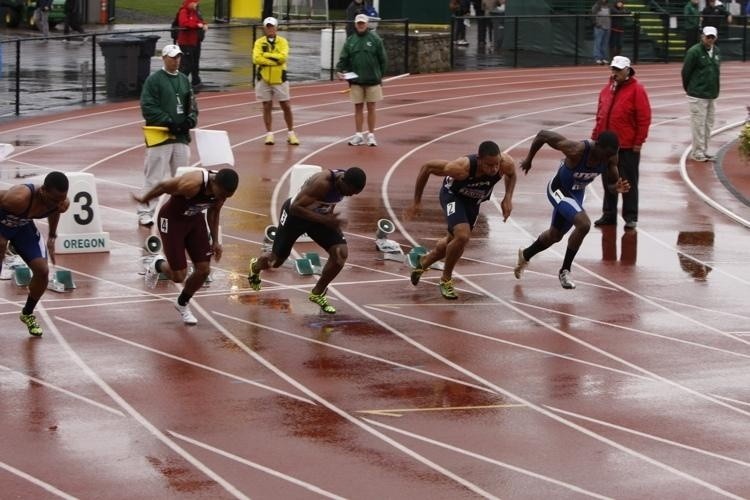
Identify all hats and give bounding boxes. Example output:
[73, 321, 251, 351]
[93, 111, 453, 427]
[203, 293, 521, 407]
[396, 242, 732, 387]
[162, 44, 184, 58]
[703, 26, 717, 37]
[354, 13, 368, 23]
[610, 55, 630, 69]
[263, 17, 278, 26]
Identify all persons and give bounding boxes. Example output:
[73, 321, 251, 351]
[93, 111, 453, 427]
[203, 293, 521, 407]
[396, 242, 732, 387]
[136, 41, 202, 227]
[513, 128, 631, 291]
[250, 16, 301, 146]
[59, 0, 89, 43]
[1, 170, 71, 337]
[601, 225, 638, 266]
[608, 0, 630, 68]
[131, 167, 240, 325]
[697, 0, 733, 61]
[31, 0, 54, 45]
[589, 0, 615, 67]
[344, 0, 378, 38]
[248, 167, 367, 316]
[333, 13, 389, 147]
[674, 232, 716, 279]
[173, 1, 209, 88]
[678, 25, 721, 165]
[590, 54, 653, 232]
[683, 0, 703, 54]
[480, 0, 506, 53]
[447, 0, 471, 46]
[409, 140, 517, 301]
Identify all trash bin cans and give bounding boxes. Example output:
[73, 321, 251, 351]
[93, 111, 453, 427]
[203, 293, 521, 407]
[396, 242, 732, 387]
[97, 33, 161, 103]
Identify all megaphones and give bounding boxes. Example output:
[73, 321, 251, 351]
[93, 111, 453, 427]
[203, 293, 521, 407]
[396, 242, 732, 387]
[143, 234, 162, 254]
[376, 218, 395, 238]
[263, 224, 277, 244]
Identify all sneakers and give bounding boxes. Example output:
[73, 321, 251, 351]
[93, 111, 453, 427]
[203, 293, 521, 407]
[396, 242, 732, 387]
[690, 152, 718, 163]
[308, 289, 336, 314]
[348, 135, 377, 146]
[174, 296, 198, 324]
[138, 214, 154, 226]
[513, 247, 529, 279]
[146, 255, 166, 290]
[248, 257, 262, 290]
[264, 134, 274, 145]
[410, 254, 430, 286]
[624, 220, 636, 231]
[559, 268, 576, 289]
[594, 212, 618, 225]
[287, 133, 299, 145]
[20, 308, 43, 336]
[440, 276, 457, 300]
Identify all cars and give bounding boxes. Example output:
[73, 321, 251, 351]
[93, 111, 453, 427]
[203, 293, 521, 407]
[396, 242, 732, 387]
[5, 0, 66, 27]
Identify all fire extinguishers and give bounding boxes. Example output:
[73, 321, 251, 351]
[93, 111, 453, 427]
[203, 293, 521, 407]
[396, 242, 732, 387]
[99, 0, 109, 24]
